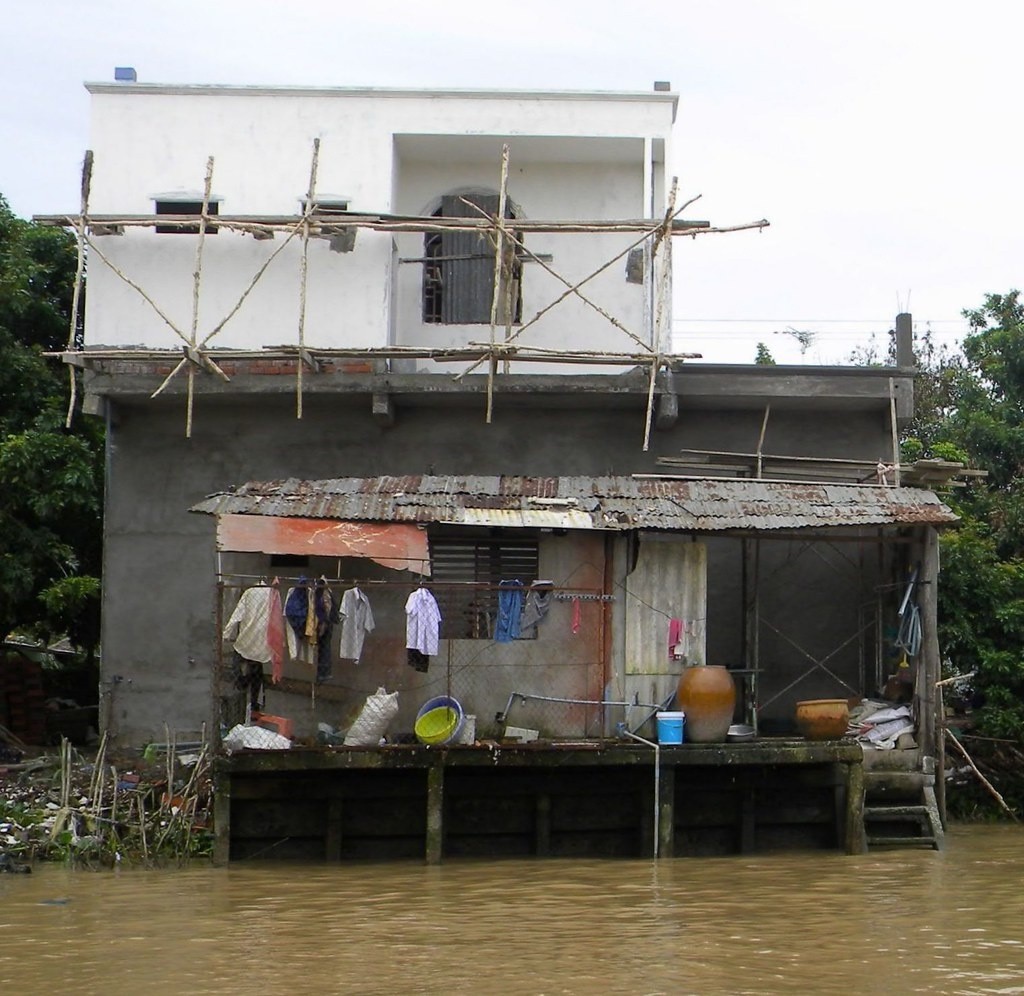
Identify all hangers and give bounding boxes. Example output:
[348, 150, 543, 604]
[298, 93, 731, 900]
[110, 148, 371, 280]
[258, 575, 424, 592]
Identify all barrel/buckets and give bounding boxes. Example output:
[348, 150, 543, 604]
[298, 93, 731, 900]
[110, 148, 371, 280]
[655, 711, 687, 745]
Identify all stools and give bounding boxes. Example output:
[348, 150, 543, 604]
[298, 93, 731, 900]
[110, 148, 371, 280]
[252, 711, 293, 740]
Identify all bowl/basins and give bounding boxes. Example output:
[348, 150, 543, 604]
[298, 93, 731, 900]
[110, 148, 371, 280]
[729, 724, 757, 743]
[415, 696, 464, 744]
[415, 706, 458, 743]
